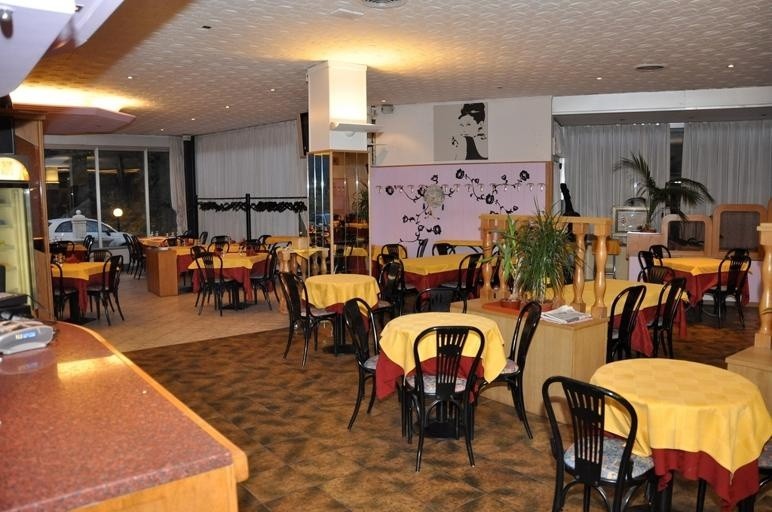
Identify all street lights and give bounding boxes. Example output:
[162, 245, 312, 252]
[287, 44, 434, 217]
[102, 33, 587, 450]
[112, 208, 123, 232]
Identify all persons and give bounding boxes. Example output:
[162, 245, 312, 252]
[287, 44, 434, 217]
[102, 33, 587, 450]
[451, 104, 488, 161]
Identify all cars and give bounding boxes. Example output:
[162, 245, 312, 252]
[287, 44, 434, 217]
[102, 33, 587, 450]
[310, 214, 330, 226]
[48, 219, 134, 250]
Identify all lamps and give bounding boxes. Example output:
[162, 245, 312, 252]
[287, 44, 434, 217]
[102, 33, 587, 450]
[45, 167, 60, 184]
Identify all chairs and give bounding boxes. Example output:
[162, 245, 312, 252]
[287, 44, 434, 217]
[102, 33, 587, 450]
[343, 297, 408, 436]
[469, 300, 543, 441]
[402, 325, 486, 474]
[542, 375, 660, 512]
[524, 244, 751, 362]
[275, 271, 341, 369]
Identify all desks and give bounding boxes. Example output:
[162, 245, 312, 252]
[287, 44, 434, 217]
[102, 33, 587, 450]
[376, 311, 508, 419]
[585, 358, 772, 512]
[0, 317, 250, 512]
